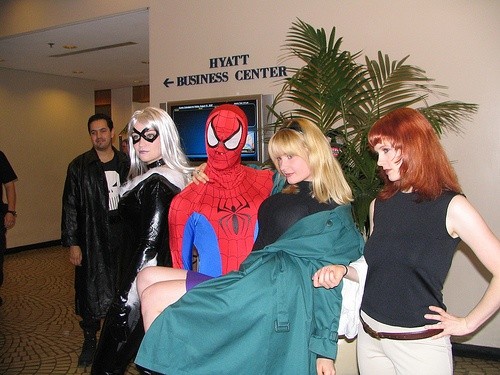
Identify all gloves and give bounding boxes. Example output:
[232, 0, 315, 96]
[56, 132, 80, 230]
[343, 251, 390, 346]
[115, 304, 130, 342]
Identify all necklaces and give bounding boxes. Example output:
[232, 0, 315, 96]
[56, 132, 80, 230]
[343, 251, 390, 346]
[147, 158, 165, 170]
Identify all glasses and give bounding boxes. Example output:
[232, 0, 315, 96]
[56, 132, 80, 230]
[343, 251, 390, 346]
[279, 121, 304, 133]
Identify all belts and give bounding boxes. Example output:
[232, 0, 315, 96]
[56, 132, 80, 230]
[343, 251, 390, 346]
[359, 308, 444, 341]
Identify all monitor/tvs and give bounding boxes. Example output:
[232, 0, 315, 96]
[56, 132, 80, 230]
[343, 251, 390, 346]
[167, 93, 263, 165]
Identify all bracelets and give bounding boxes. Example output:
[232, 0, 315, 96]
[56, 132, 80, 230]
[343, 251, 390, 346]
[338, 264, 348, 277]
[7, 210, 16, 216]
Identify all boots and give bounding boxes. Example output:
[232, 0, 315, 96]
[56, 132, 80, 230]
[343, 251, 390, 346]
[79, 323, 97, 366]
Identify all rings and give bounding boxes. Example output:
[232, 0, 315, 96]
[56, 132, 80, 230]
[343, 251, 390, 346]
[197, 170, 200, 173]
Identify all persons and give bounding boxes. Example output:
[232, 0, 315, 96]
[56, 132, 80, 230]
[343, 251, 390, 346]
[0, 150, 18, 287]
[90, 108, 197, 375]
[310, 107, 500, 375]
[135, 118, 366, 375]
[166, 104, 276, 279]
[61, 114, 131, 368]
